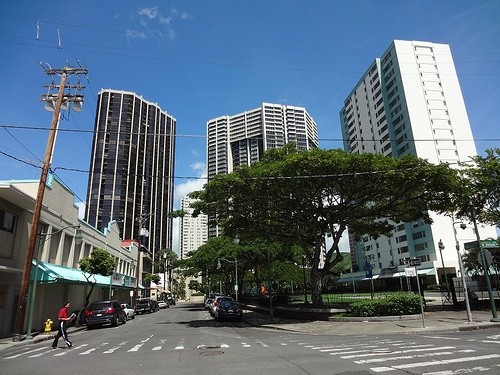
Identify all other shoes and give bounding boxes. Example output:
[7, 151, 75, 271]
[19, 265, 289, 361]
[67, 344, 75, 349]
[50, 346, 60, 350]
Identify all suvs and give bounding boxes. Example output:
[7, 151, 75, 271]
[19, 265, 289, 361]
[84, 300, 127, 330]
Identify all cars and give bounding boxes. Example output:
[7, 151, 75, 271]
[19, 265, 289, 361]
[134, 298, 177, 315]
[121, 303, 136, 321]
[214, 300, 242, 322]
[204, 295, 235, 317]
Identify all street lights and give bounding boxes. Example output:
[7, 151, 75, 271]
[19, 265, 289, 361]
[437, 238, 451, 299]
[451, 216, 474, 323]
[11, 223, 81, 343]
[217, 256, 239, 301]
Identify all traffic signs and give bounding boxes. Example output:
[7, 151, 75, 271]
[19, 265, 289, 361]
[407, 258, 422, 267]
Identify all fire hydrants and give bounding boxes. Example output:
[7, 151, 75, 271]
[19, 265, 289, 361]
[44, 319, 53, 335]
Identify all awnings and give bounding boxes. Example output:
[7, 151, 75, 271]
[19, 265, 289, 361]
[31, 258, 146, 290]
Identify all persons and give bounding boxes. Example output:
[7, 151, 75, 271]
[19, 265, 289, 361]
[50, 301, 75, 350]
[257, 283, 266, 308]
